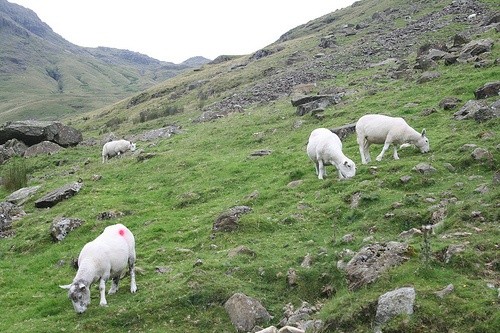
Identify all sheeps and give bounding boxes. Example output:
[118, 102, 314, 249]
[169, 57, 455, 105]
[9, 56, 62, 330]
[102, 140, 136, 164]
[59, 223, 138, 314]
[355, 113, 430, 165]
[307, 127, 357, 180]
